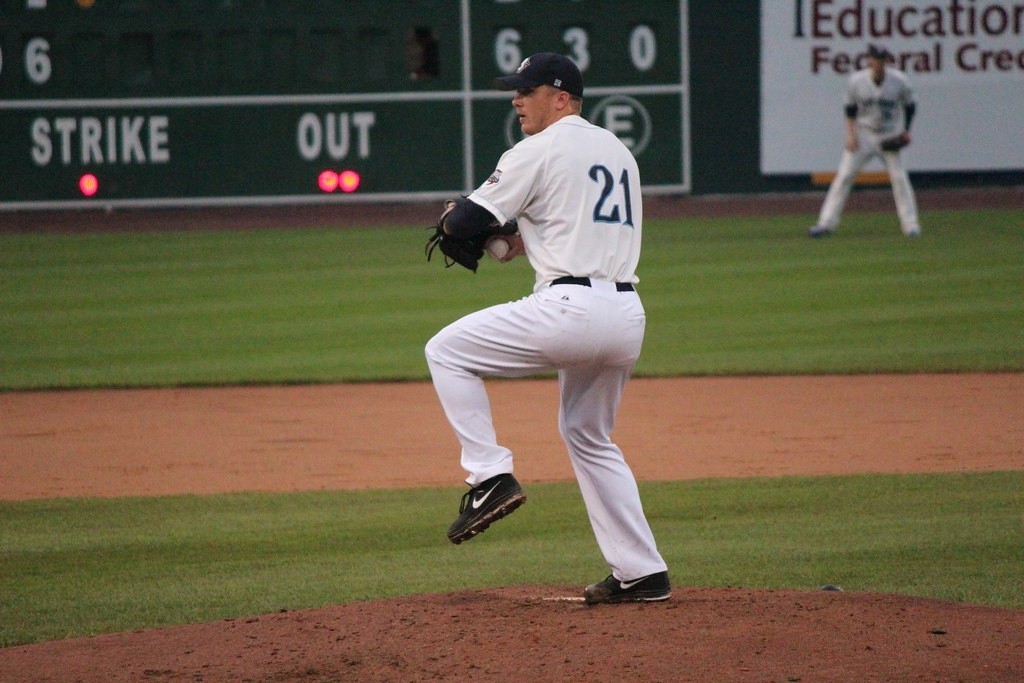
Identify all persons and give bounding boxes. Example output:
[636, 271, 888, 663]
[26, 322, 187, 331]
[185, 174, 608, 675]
[425, 53, 671, 604]
[808, 45, 922, 239]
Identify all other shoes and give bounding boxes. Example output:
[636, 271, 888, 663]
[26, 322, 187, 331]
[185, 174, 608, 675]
[905, 228, 921, 237]
[807, 225, 830, 236]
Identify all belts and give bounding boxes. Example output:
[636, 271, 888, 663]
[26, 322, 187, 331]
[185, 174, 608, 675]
[550, 277, 634, 291]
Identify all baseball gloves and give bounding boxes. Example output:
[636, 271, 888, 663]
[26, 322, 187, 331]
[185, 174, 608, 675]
[436, 199, 486, 271]
[880, 135, 908, 151]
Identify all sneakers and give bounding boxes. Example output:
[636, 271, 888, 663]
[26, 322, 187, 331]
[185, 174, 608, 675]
[584, 573, 671, 604]
[447, 474, 526, 546]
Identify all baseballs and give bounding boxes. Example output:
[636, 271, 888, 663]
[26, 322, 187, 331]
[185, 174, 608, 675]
[489, 238, 509, 260]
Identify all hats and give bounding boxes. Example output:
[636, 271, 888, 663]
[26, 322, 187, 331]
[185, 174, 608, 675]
[867, 46, 886, 58]
[492, 53, 584, 99]
